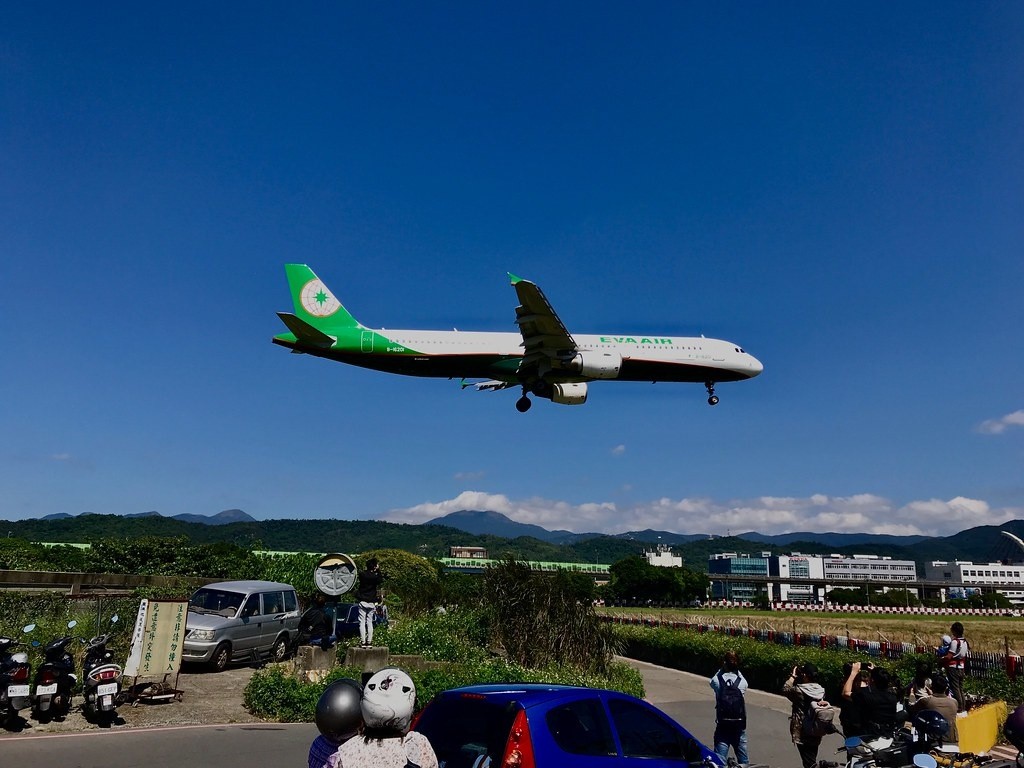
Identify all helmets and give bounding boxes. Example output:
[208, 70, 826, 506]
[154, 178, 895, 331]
[914, 710, 950, 736]
[361, 666, 416, 725]
[1003, 704, 1024, 755]
[315, 679, 364, 743]
[11, 653, 28, 663]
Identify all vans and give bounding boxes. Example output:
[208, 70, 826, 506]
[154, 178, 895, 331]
[176, 580, 300, 671]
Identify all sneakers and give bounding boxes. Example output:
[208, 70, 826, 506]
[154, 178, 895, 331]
[359, 641, 372, 648]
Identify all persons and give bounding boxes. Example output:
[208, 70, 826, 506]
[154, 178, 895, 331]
[837, 661, 867, 708]
[781, 662, 826, 768]
[306, 678, 364, 768]
[903, 661, 932, 699]
[354, 557, 382, 648]
[838, 667, 896, 761]
[708, 651, 748, 765]
[309, 590, 338, 649]
[938, 622, 969, 713]
[320, 666, 439, 768]
[904, 676, 959, 743]
[932, 635, 952, 657]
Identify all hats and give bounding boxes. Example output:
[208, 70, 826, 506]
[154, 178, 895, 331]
[941, 635, 952, 645]
[799, 664, 818, 676]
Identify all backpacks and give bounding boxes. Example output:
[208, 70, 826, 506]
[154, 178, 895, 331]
[716, 674, 747, 731]
[802, 691, 834, 735]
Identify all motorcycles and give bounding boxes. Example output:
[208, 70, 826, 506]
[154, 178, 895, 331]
[79, 615, 123, 723]
[810, 715, 914, 768]
[691, 754, 769, 767]
[31, 620, 77, 720]
[0, 624, 36, 727]
[924, 717, 992, 768]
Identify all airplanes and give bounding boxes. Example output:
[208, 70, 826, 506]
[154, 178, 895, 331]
[271, 264, 764, 412]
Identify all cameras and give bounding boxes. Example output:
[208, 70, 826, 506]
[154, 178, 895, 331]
[796, 667, 804, 676]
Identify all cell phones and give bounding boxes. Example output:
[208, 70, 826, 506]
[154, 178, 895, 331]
[861, 663, 871, 670]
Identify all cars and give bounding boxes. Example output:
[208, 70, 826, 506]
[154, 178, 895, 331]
[300, 603, 388, 648]
[414, 683, 729, 768]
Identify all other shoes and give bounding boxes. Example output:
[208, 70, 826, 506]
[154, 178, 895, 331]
[957, 711, 967, 717]
